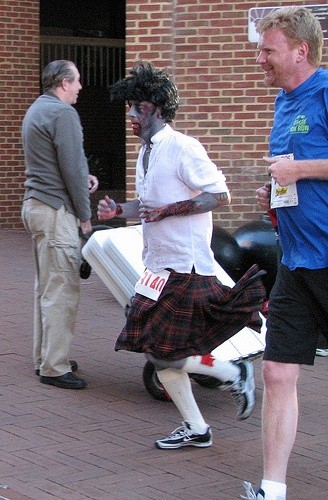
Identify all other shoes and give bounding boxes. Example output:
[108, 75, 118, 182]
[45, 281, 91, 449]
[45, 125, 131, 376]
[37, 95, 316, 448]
[40, 372, 88, 390]
[35, 360, 78, 376]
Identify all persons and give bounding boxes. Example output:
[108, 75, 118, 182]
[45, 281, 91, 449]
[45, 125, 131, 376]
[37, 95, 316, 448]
[96, 62, 266, 447]
[244, 7, 328, 500]
[22, 60, 99, 388]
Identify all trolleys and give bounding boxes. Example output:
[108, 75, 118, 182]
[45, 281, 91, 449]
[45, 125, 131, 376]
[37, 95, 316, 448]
[77, 224, 266, 402]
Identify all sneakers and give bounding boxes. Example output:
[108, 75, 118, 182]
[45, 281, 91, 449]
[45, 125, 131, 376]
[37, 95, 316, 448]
[155, 421, 212, 450]
[238, 480, 286, 500]
[218, 361, 256, 421]
